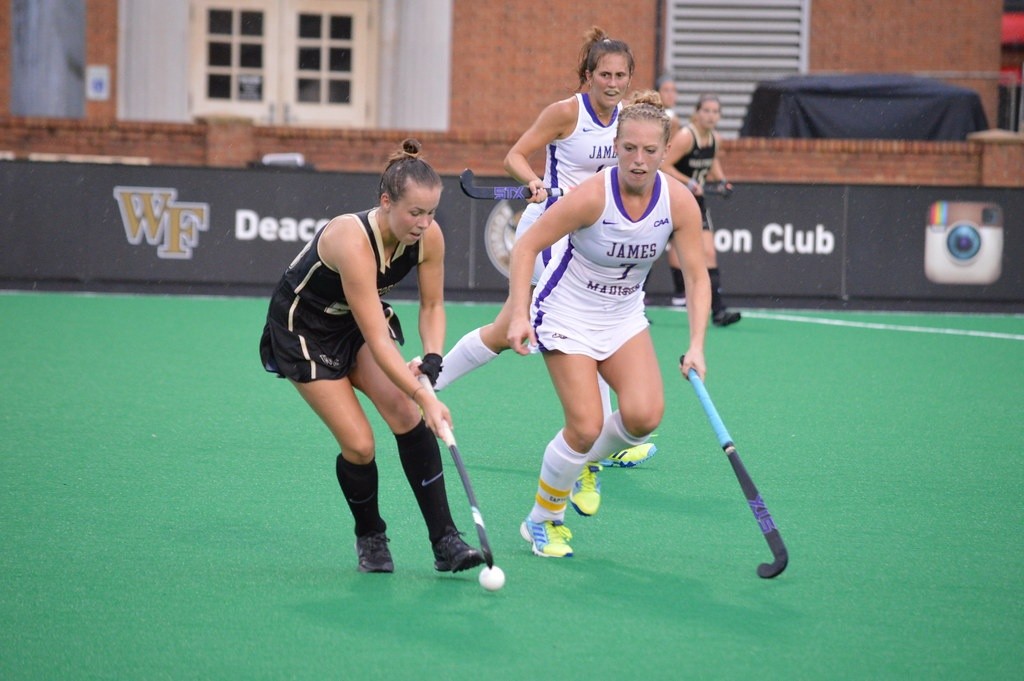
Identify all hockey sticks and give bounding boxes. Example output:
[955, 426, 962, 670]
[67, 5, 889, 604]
[459, 167, 571, 201]
[679, 354, 790, 580]
[689, 182, 735, 198]
[411, 356, 495, 571]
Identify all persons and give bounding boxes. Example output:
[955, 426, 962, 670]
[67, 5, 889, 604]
[259, 138, 484, 574]
[408, 26, 741, 557]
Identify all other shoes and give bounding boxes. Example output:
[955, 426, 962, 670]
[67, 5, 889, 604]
[671, 293, 686, 306]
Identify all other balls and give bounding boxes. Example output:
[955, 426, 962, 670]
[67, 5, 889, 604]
[479, 565, 506, 591]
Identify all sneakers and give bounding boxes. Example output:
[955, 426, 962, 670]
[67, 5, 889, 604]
[416, 404, 424, 417]
[713, 311, 741, 326]
[355, 532, 394, 573]
[600, 443, 658, 468]
[434, 526, 484, 573]
[520, 515, 573, 558]
[570, 462, 604, 516]
